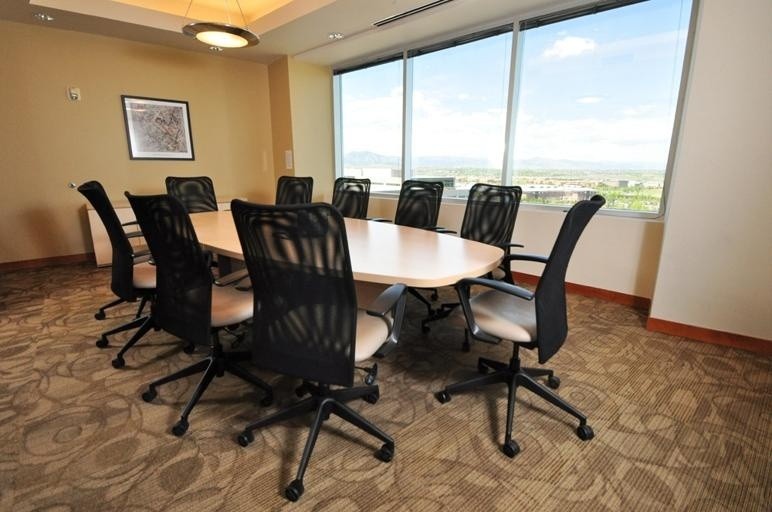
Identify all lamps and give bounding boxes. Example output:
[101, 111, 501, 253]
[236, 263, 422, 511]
[181, 0, 259, 49]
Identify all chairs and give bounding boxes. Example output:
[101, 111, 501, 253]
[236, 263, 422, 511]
[230, 200, 395, 502]
[123, 190, 274, 436]
[421, 183, 522, 353]
[331, 178, 370, 218]
[275, 176, 313, 205]
[75, 183, 213, 367]
[372, 179, 446, 314]
[435, 194, 605, 456]
[166, 175, 218, 212]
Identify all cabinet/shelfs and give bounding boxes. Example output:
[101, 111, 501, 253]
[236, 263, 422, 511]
[86, 194, 249, 269]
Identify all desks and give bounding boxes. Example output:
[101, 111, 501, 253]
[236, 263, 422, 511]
[188, 209, 506, 335]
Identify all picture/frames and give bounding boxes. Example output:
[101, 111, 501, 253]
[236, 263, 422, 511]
[120, 95, 195, 161]
